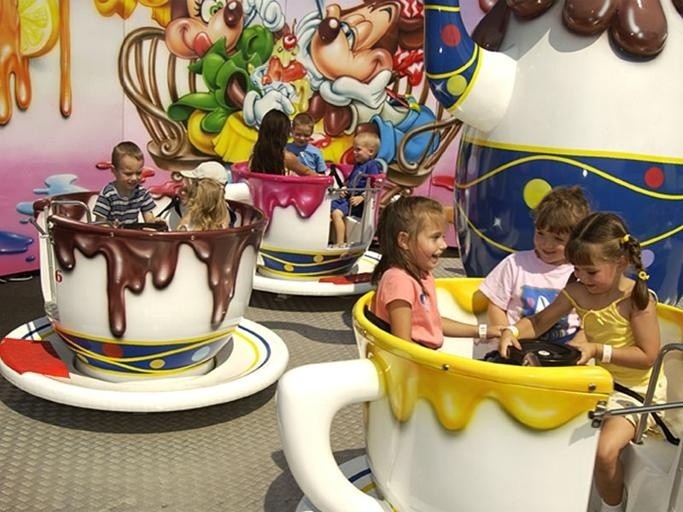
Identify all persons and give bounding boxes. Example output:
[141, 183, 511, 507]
[331, 132, 380, 245]
[93, 141, 163, 227]
[480, 185, 593, 341]
[508, 212, 667, 512]
[178, 160, 236, 228]
[178, 178, 230, 229]
[285, 112, 327, 175]
[246, 109, 327, 179]
[371, 193, 504, 361]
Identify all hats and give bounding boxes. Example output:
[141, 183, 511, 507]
[177, 161, 230, 187]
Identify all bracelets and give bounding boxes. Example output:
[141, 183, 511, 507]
[601, 344, 614, 365]
[362, 191, 368, 198]
[479, 324, 490, 342]
[506, 325, 522, 336]
